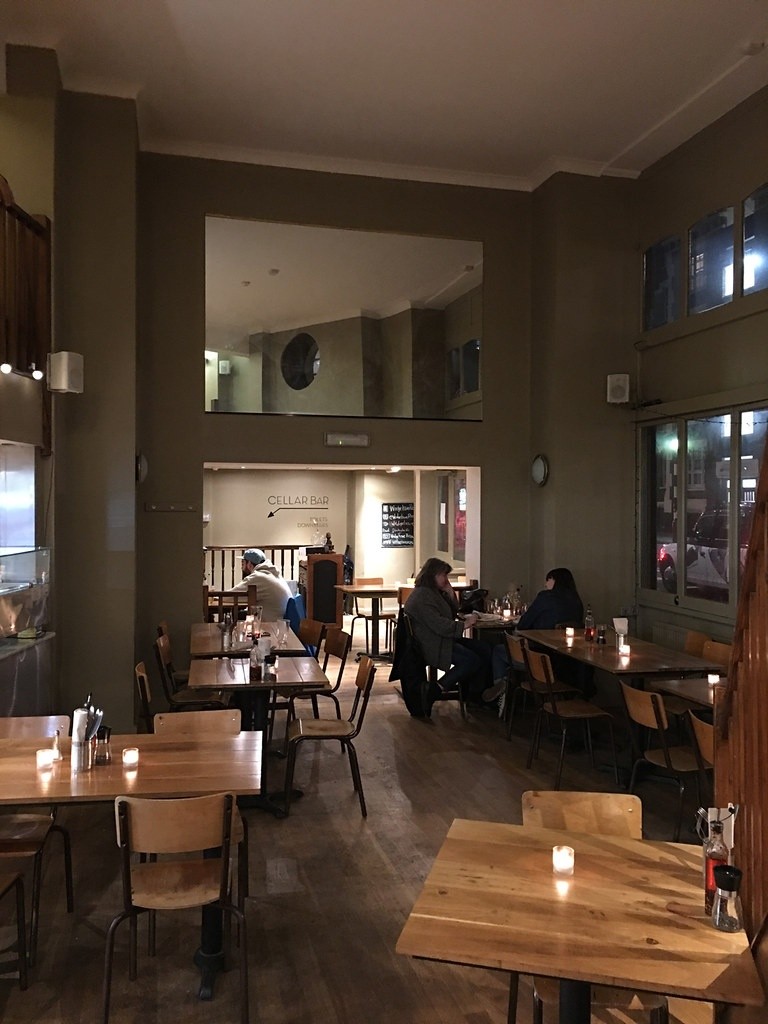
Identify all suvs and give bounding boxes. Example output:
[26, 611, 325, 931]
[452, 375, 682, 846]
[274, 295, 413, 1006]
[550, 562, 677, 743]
[658, 501, 756, 594]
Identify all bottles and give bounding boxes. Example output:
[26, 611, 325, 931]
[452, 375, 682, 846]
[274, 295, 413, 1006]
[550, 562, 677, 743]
[264, 656, 276, 681]
[487, 593, 526, 615]
[252, 612, 260, 643]
[53, 730, 63, 761]
[598, 624, 607, 643]
[585, 609, 594, 641]
[250, 640, 262, 681]
[93, 725, 111, 765]
[704, 822, 728, 915]
[710, 866, 743, 933]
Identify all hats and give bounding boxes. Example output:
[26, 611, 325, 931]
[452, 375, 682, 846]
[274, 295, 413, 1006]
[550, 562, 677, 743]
[240, 549, 266, 566]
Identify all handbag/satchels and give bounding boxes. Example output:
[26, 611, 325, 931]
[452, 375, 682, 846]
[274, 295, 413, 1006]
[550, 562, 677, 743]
[458, 588, 488, 614]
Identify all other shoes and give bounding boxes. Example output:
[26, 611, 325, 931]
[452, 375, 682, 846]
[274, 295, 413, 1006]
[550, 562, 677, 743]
[482, 680, 506, 719]
[465, 702, 498, 713]
[422, 682, 436, 718]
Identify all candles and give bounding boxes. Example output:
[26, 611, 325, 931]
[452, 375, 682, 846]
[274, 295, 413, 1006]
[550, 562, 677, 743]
[552, 845, 575, 876]
[503, 610, 511, 617]
[566, 627, 574, 638]
[122, 747, 139, 769]
[36, 748, 52, 772]
[619, 645, 630, 656]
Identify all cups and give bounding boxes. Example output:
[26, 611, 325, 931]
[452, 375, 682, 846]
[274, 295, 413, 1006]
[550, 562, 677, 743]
[237, 621, 248, 642]
[71, 740, 92, 771]
[222, 635, 232, 652]
[552, 846, 574, 877]
[616, 633, 627, 654]
[36, 749, 52, 769]
[276, 620, 289, 644]
[123, 748, 139, 770]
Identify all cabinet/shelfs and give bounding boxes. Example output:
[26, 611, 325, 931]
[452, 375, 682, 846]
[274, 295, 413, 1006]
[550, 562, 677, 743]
[307, 554, 345, 639]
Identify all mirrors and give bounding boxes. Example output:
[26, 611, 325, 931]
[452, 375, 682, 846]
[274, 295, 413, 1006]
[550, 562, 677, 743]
[203, 211, 485, 423]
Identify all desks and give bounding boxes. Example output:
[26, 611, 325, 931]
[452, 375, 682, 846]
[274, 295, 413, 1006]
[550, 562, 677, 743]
[333, 583, 471, 664]
[392, 818, 764, 1024]
[1, 731, 264, 1001]
[190, 621, 308, 757]
[189, 657, 334, 820]
[515, 627, 727, 797]
[648, 675, 730, 835]
[209, 600, 250, 623]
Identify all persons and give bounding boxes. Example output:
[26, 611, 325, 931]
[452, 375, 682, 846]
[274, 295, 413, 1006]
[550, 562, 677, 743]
[492, 568, 586, 678]
[217, 547, 294, 623]
[404, 557, 484, 697]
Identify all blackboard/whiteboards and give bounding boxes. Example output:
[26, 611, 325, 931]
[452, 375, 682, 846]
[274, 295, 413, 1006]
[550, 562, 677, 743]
[381, 502, 415, 548]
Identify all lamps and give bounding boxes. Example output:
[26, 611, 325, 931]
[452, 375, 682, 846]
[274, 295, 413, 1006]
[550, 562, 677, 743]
[530, 454, 550, 488]
[135, 450, 149, 485]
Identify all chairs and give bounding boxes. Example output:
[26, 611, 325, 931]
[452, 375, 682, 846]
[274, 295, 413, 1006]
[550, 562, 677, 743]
[0, 579, 670, 1024]
